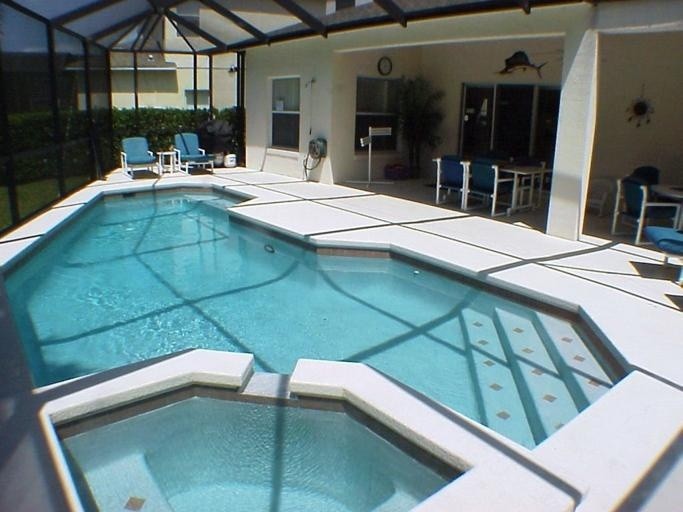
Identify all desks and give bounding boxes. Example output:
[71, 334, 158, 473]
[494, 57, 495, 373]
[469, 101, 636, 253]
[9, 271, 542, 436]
[156, 151, 176, 175]
[651, 183, 683, 232]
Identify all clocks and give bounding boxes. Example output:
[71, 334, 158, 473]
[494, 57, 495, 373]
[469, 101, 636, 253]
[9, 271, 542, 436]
[378, 57, 392, 75]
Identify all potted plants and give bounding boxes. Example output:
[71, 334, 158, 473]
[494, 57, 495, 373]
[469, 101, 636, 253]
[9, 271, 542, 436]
[389, 69, 446, 180]
[197, 119, 233, 167]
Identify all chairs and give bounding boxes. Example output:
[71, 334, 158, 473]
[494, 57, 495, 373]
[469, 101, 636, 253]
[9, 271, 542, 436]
[120, 137, 159, 179]
[589, 178, 612, 218]
[433, 151, 552, 218]
[630, 166, 675, 235]
[173, 133, 216, 175]
[645, 225, 683, 286]
[612, 176, 680, 246]
